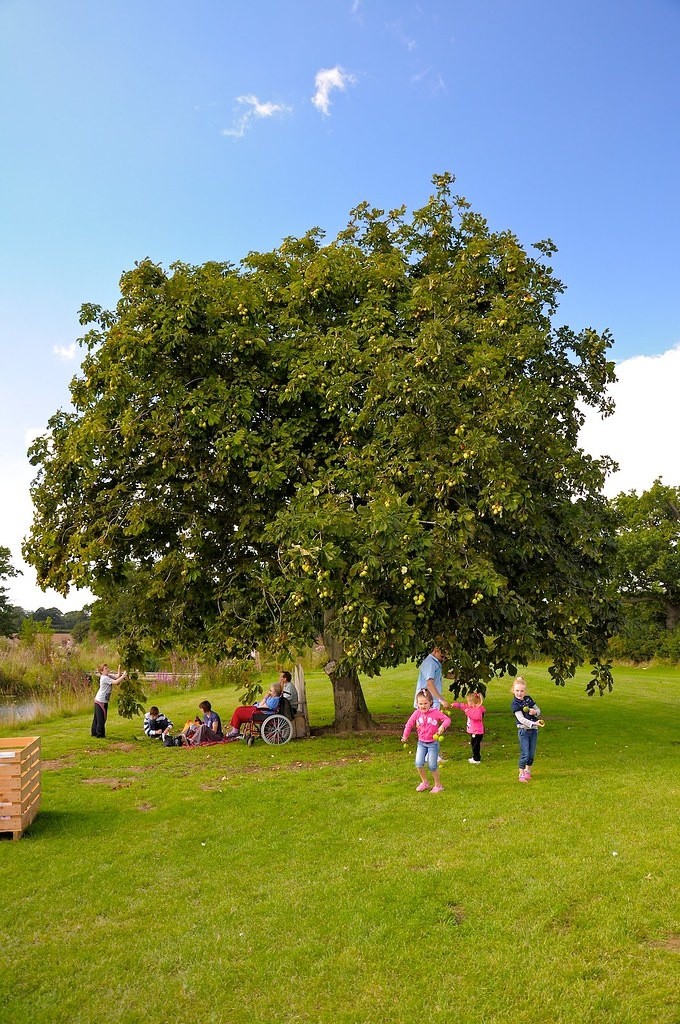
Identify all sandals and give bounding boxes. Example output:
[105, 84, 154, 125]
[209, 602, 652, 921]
[430, 784, 444, 793]
[416, 780, 430, 791]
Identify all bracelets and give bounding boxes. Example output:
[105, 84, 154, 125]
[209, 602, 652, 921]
[439, 698, 445, 704]
[264, 697, 267, 700]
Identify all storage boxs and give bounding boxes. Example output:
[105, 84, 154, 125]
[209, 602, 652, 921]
[0, 736, 42, 841]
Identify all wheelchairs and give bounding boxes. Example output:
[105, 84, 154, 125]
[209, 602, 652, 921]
[241, 691, 294, 747]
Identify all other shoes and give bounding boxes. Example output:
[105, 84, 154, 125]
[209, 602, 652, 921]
[226, 731, 239, 738]
[468, 757, 480, 764]
[183, 733, 192, 746]
[425, 752, 448, 763]
[524, 769, 532, 780]
[519, 773, 528, 782]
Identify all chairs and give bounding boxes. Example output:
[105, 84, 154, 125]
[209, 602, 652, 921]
[282, 664, 310, 738]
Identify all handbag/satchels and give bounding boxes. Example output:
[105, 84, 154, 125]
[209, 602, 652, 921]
[165, 735, 182, 746]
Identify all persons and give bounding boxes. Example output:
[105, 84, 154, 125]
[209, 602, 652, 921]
[226, 669, 299, 738]
[91, 662, 127, 738]
[178, 701, 223, 746]
[509, 676, 546, 787]
[449, 692, 485, 765]
[400, 688, 451, 795]
[414, 647, 449, 762]
[144, 706, 174, 740]
